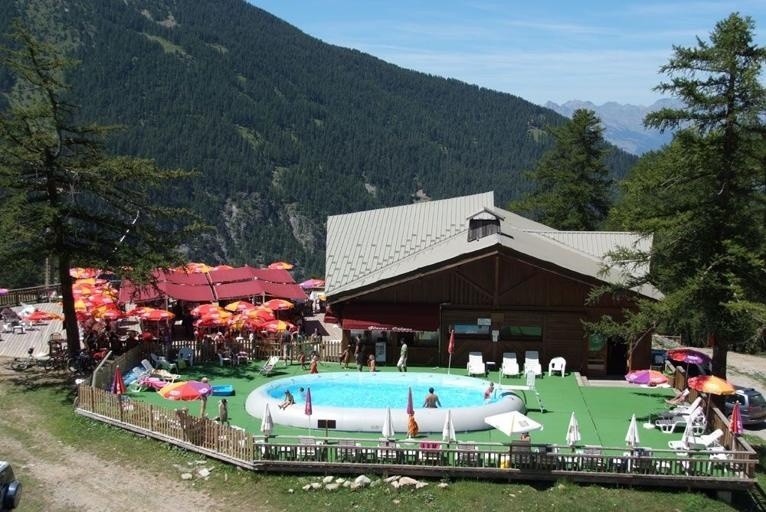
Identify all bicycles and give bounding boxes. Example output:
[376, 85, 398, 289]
[10, 344, 95, 377]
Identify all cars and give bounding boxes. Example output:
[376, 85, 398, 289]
[650, 346, 666, 368]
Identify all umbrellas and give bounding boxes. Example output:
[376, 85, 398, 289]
[728, 400, 744, 450]
[688, 375, 735, 419]
[382, 405, 395, 439]
[484, 410, 544, 441]
[624, 414, 640, 447]
[566, 411, 581, 446]
[442, 408, 456, 442]
[407, 385, 414, 437]
[157, 380, 212, 401]
[624, 370, 669, 422]
[110, 365, 128, 395]
[304, 384, 312, 436]
[448, 330, 455, 374]
[667, 349, 712, 388]
[681, 416, 695, 450]
[260, 402, 274, 441]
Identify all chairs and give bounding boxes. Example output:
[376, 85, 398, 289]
[178, 347, 194, 366]
[548, 356, 566, 378]
[499, 352, 520, 379]
[524, 350, 542, 379]
[132, 352, 177, 393]
[297, 435, 653, 468]
[655, 389, 724, 449]
[466, 351, 486, 378]
[217, 351, 248, 368]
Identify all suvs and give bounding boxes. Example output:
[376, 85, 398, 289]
[0, 460, 21, 512]
[709, 385, 765, 428]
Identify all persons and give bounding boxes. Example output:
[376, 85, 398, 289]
[278, 390, 294, 410]
[484, 382, 495, 399]
[218, 399, 229, 424]
[200, 377, 208, 419]
[194, 327, 241, 368]
[339, 334, 375, 372]
[520, 432, 531, 442]
[80, 316, 172, 358]
[281, 323, 318, 374]
[421, 387, 441, 408]
[397, 338, 408, 372]
